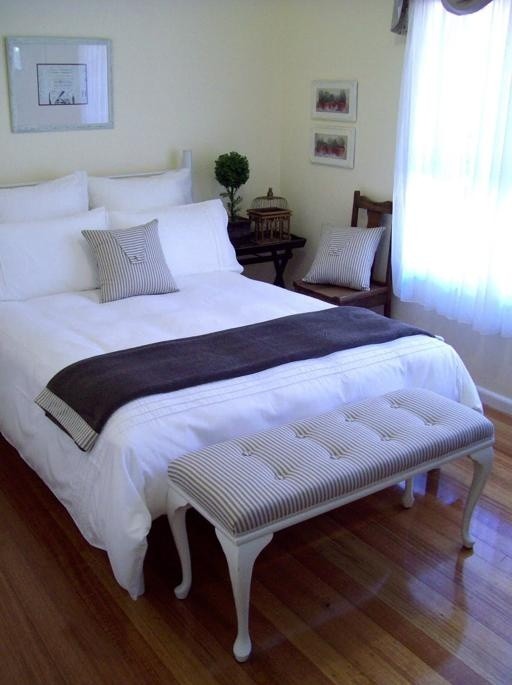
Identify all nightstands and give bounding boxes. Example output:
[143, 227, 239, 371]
[228, 229, 307, 287]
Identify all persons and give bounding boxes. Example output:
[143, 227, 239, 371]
[314, 141, 346, 157]
[317, 97, 348, 114]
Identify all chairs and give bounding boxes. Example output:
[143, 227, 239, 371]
[292, 188, 392, 319]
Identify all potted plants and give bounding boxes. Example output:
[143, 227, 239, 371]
[213, 152, 252, 242]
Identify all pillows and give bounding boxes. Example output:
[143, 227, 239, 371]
[0, 206, 111, 301]
[106, 198, 244, 274]
[80, 219, 180, 303]
[87, 168, 195, 210]
[301, 220, 386, 293]
[0, 170, 89, 219]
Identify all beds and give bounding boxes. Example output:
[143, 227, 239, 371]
[1, 268, 485, 602]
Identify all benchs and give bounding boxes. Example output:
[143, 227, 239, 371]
[165, 386, 497, 662]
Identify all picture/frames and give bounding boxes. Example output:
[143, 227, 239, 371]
[310, 78, 358, 123]
[307, 124, 355, 169]
[4, 35, 114, 133]
